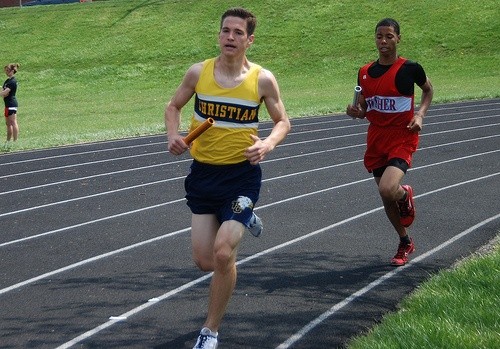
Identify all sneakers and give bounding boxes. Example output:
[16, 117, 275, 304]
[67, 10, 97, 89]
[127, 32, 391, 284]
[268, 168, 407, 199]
[390, 236, 416, 266]
[397, 184, 416, 229]
[246, 211, 264, 238]
[193, 327, 219, 349]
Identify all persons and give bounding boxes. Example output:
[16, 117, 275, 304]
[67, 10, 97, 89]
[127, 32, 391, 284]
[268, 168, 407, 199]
[1, 62, 21, 141]
[165, 7, 291, 349]
[346, 18, 435, 265]
